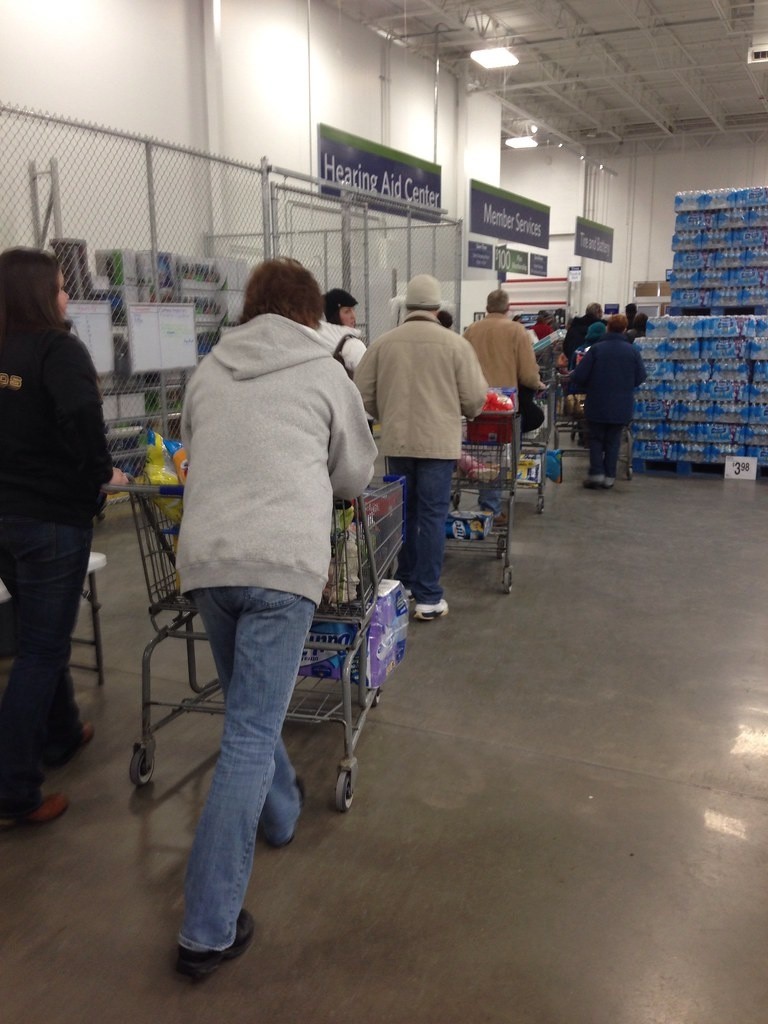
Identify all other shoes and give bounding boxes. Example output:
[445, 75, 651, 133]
[81, 724, 96, 743]
[494, 513, 507, 526]
[270, 776, 304, 845]
[174, 908, 254, 977]
[0, 794, 67, 829]
[603, 476, 615, 489]
[582, 473, 605, 489]
[413, 598, 449, 620]
[406, 590, 414, 596]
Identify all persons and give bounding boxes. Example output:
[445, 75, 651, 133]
[513, 316, 522, 322]
[562, 303, 648, 488]
[176, 257, 379, 979]
[319, 287, 374, 434]
[532, 310, 557, 404]
[437, 310, 453, 328]
[354, 274, 487, 619]
[0, 247, 114, 832]
[463, 290, 547, 524]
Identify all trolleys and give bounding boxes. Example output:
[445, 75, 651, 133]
[102, 474, 410, 814]
[383, 386, 521, 596]
[519, 343, 634, 515]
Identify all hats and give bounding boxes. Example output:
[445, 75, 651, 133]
[537, 310, 552, 322]
[405, 275, 443, 310]
[584, 322, 606, 339]
[324, 289, 358, 324]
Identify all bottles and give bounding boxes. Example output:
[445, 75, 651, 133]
[669, 184, 768, 306]
[631, 315, 768, 462]
